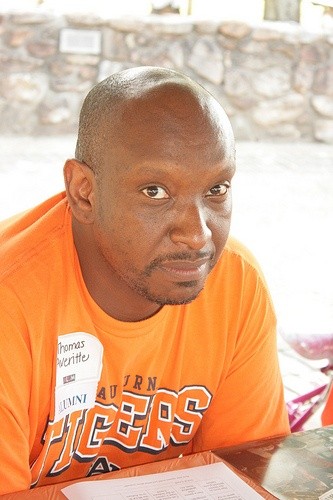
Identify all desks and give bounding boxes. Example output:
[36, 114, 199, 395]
[0, 421, 333, 498]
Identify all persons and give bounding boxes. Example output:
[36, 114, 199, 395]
[0, 65, 294, 498]
[261, 438, 333, 490]
